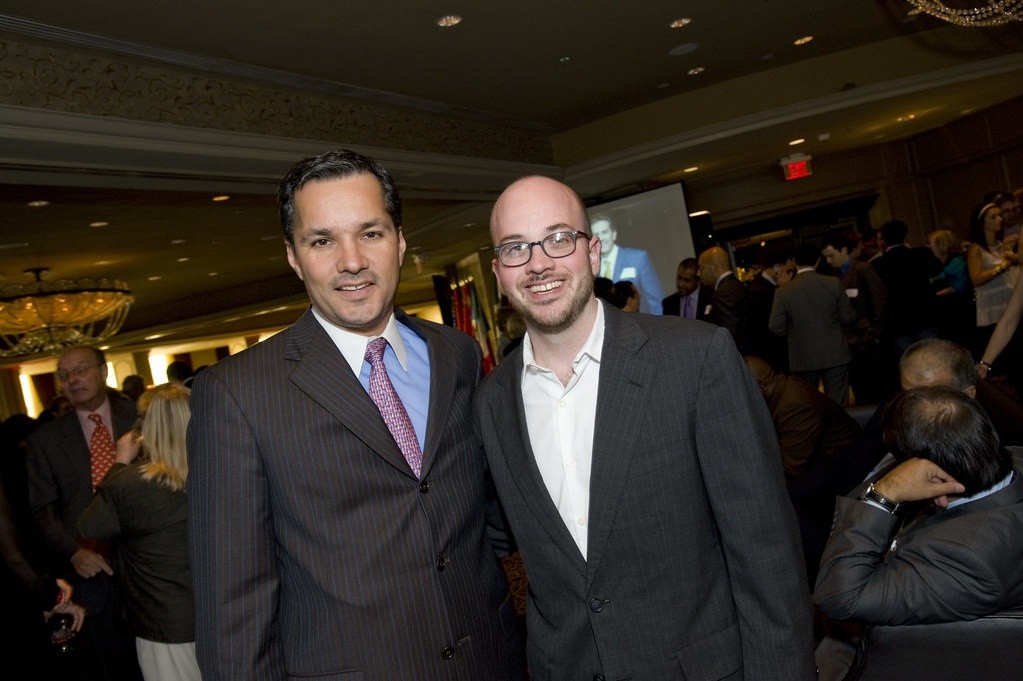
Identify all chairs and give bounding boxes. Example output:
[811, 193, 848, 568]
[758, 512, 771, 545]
[839, 612, 1023, 681]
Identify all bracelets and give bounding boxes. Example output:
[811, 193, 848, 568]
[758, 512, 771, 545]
[980, 359, 993, 371]
[863, 482, 900, 515]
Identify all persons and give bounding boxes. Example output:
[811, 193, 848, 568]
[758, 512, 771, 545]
[468, 176, 814, 680]
[187, 153, 521, 681]
[591, 217, 665, 317]
[661, 191, 1023, 681]
[616, 281, 641, 313]
[0, 347, 212, 681]
[594, 277, 615, 304]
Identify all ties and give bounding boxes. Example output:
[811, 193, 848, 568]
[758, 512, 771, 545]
[604, 260, 611, 280]
[364, 337, 423, 480]
[88, 413, 116, 495]
[683, 296, 694, 319]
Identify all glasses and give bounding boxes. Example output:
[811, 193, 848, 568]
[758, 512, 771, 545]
[57, 364, 101, 382]
[494, 230, 591, 266]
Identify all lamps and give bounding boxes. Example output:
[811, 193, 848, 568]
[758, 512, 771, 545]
[0, 266, 135, 359]
